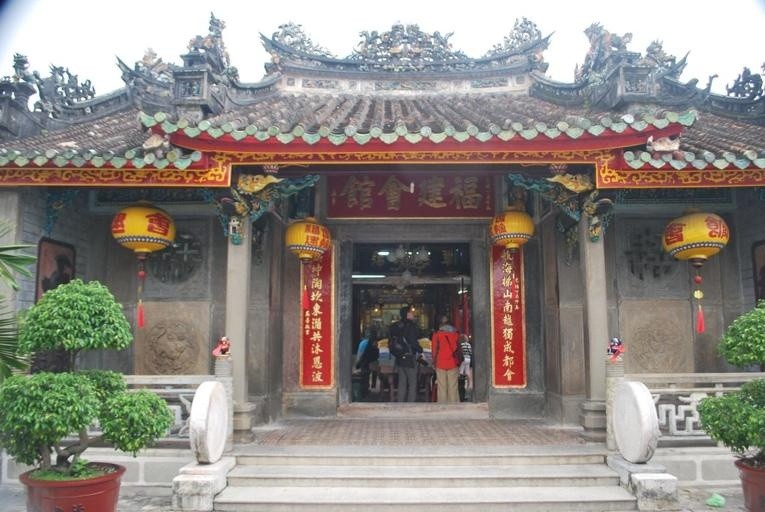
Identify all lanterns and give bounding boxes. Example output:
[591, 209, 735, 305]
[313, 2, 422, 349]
[660, 208, 731, 332]
[489, 206, 534, 303]
[286, 215, 333, 311]
[111, 200, 176, 326]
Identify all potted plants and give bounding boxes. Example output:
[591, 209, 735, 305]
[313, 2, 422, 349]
[2, 274, 171, 512]
[696, 297, 764, 511]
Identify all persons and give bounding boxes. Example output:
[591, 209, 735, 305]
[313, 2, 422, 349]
[356, 331, 371, 372]
[459, 334, 474, 391]
[364, 335, 380, 388]
[431, 315, 462, 403]
[390, 306, 423, 403]
